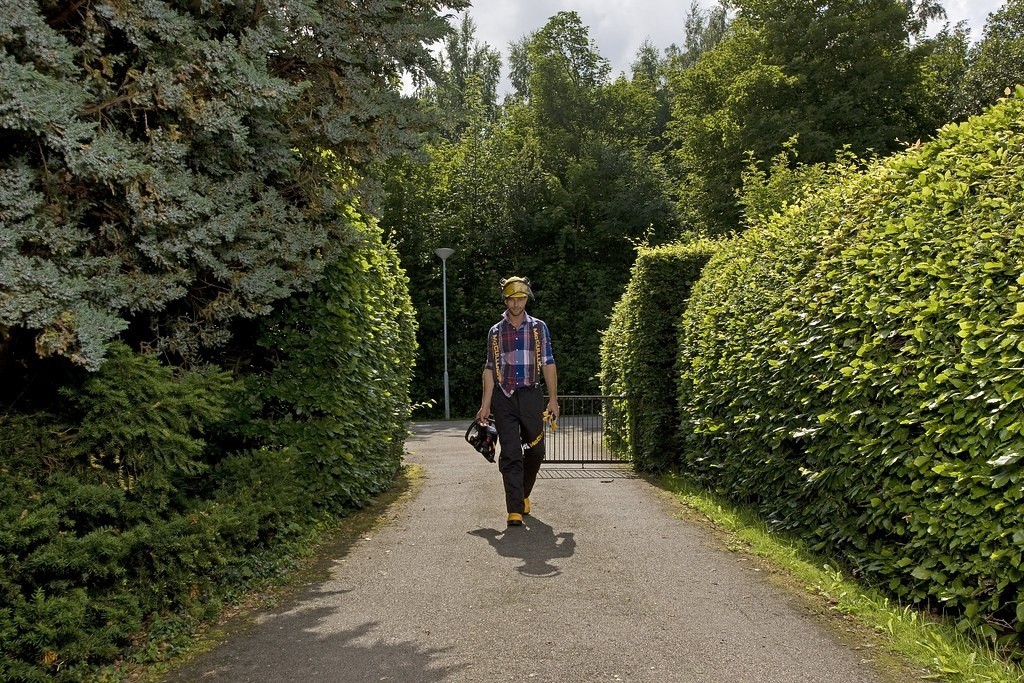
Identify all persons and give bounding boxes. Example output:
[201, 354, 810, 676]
[475, 276, 560, 525]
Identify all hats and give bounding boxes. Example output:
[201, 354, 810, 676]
[500, 276, 530, 297]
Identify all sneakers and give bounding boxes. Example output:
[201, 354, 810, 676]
[507, 512, 522, 527]
[524, 498, 530, 515]
